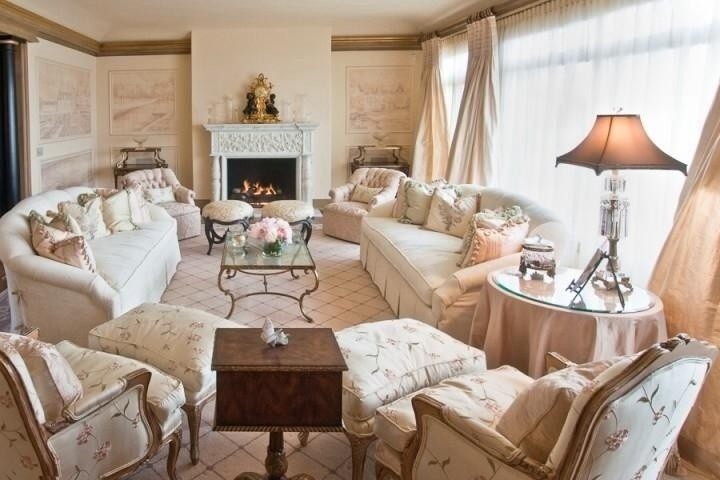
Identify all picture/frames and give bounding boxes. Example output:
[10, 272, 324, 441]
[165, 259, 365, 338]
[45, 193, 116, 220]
[35, 55, 95, 146]
[109, 145, 183, 184]
[345, 64, 416, 135]
[345, 145, 413, 177]
[107, 69, 179, 137]
[37, 148, 97, 192]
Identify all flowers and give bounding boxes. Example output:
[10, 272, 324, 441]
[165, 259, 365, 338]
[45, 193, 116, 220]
[246, 215, 294, 246]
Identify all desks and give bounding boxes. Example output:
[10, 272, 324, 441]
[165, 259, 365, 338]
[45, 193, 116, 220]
[209, 325, 349, 480]
[468, 264, 669, 381]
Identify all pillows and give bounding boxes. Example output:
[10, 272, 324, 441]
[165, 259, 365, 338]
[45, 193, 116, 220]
[492, 354, 627, 463]
[28, 189, 152, 273]
[392, 176, 531, 269]
[350, 183, 384, 204]
[1, 332, 82, 421]
[141, 185, 177, 205]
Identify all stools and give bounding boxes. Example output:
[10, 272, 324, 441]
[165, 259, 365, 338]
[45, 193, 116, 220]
[296, 316, 489, 480]
[260, 199, 314, 245]
[87, 300, 251, 465]
[201, 199, 255, 256]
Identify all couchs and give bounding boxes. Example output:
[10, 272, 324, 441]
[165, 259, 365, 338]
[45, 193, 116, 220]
[358, 181, 578, 346]
[1, 186, 183, 348]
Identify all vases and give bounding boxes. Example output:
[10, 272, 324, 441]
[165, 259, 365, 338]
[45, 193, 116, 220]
[262, 240, 283, 257]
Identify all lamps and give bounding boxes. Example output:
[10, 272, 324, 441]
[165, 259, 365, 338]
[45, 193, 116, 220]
[553, 107, 691, 290]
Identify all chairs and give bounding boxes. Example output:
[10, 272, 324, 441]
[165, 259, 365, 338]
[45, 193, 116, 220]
[121, 166, 203, 241]
[319, 167, 408, 245]
[1, 329, 187, 478]
[372, 334, 719, 480]
